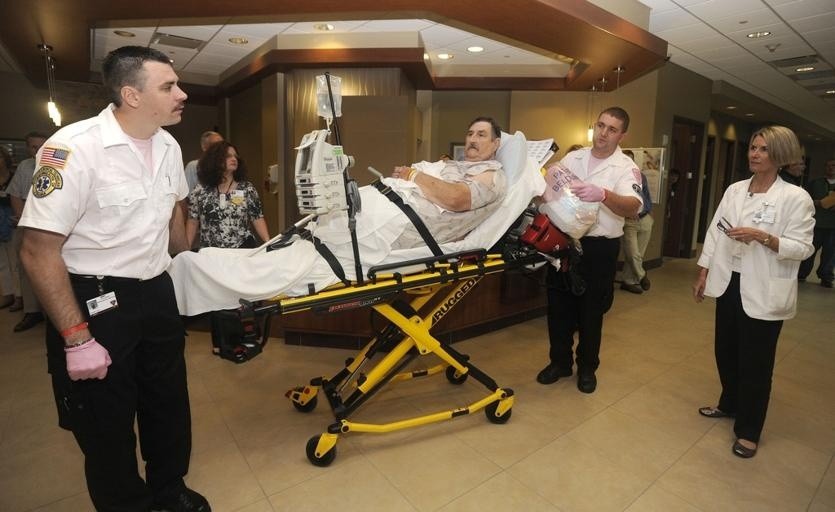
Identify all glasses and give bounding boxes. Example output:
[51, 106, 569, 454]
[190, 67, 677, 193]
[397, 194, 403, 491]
[717, 217, 735, 238]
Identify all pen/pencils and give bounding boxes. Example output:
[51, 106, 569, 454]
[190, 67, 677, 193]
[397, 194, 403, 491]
[722, 217, 749, 245]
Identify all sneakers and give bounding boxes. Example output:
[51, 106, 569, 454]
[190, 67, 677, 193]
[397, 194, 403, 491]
[697, 402, 735, 419]
[149, 466, 212, 510]
[1, 293, 43, 335]
[619, 274, 653, 296]
[733, 430, 759, 459]
[537, 358, 573, 385]
[577, 362, 597, 394]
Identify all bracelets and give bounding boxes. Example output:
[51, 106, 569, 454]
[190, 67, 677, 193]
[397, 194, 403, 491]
[57, 321, 90, 336]
[404, 168, 420, 183]
[763, 235, 773, 247]
[603, 187, 609, 203]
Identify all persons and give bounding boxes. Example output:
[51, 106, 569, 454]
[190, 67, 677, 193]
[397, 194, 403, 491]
[186, 140, 272, 361]
[620, 150, 654, 294]
[779, 155, 813, 199]
[177, 116, 508, 298]
[661, 168, 681, 262]
[535, 106, 646, 394]
[12, 44, 213, 512]
[797, 158, 834, 288]
[692, 126, 817, 458]
[6, 132, 50, 332]
[1, 144, 21, 313]
[185, 129, 224, 190]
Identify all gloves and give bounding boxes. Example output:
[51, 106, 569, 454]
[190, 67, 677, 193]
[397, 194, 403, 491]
[569, 182, 607, 204]
[63, 336, 113, 382]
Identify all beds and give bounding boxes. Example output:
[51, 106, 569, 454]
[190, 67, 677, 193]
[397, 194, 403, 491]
[165, 128, 557, 468]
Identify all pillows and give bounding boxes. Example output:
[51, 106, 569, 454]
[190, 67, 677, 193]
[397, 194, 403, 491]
[495, 130, 528, 186]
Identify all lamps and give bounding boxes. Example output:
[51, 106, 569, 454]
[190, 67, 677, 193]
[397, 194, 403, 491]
[38, 44, 62, 127]
